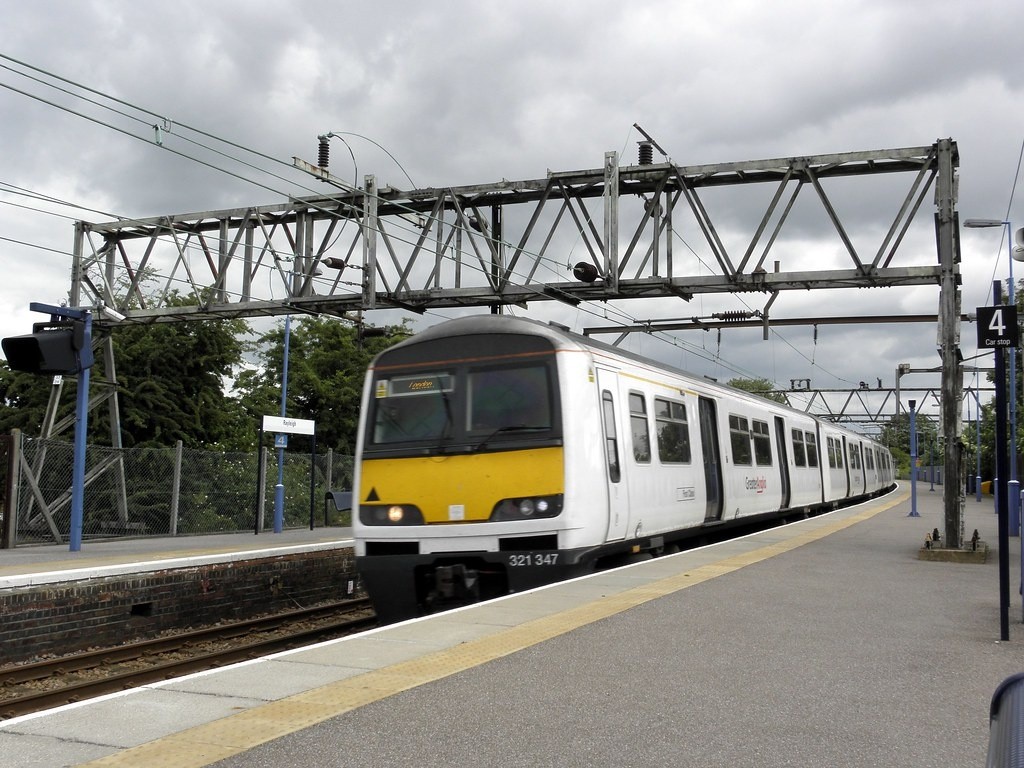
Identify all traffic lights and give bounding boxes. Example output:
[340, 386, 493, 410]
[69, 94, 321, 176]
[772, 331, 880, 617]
[2, 329, 79, 376]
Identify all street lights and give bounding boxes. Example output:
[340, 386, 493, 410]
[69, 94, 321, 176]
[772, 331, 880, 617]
[963, 214, 1018, 639]
[916, 427, 936, 491]
[270, 267, 324, 530]
[907, 398, 923, 517]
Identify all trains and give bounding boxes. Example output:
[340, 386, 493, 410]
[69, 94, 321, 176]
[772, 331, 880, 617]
[348, 313, 900, 628]
